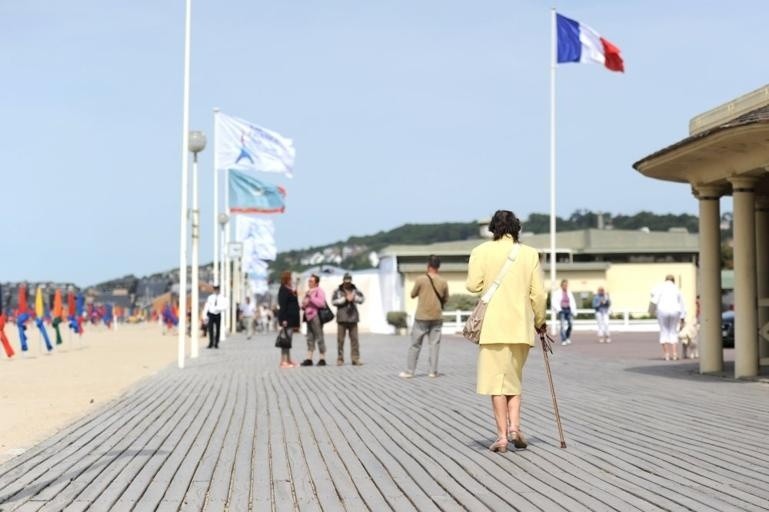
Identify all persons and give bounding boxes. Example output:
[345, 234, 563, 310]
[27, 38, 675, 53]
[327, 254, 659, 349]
[551, 279, 577, 345]
[301, 275, 327, 366]
[593, 287, 612, 343]
[651, 276, 701, 361]
[465, 209, 548, 452]
[237, 297, 298, 339]
[277, 270, 300, 368]
[331, 273, 365, 366]
[202, 286, 228, 350]
[399, 255, 449, 378]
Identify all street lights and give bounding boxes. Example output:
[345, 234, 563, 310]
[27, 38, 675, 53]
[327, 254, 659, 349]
[217, 212, 230, 341]
[186, 130, 207, 361]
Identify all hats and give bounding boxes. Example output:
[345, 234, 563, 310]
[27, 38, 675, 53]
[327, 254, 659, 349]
[344, 273, 352, 278]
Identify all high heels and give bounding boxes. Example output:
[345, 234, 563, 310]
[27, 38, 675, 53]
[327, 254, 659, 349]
[489, 436, 508, 452]
[509, 425, 527, 448]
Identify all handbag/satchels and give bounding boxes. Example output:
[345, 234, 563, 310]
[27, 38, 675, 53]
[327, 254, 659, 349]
[275, 336, 291, 348]
[318, 306, 333, 324]
[463, 299, 486, 344]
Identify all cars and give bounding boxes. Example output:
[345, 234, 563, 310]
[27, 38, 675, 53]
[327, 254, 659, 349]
[720, 309, 736, 349]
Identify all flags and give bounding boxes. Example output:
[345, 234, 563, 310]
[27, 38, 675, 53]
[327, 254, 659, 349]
[213, 111, 296, 178]
[225, 169, 287, 215]
[552, 12, 624, 73]
[236, 212, 277, 296]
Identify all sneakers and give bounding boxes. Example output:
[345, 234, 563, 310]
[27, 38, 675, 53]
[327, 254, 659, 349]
[399, 371, 412, 378]
[301, 359, 313, 365]
[352, 360, 363, 365]
[428, 373, 439, 377]
[337, 359, 343, 365]
[317, 360, 326, 365]
